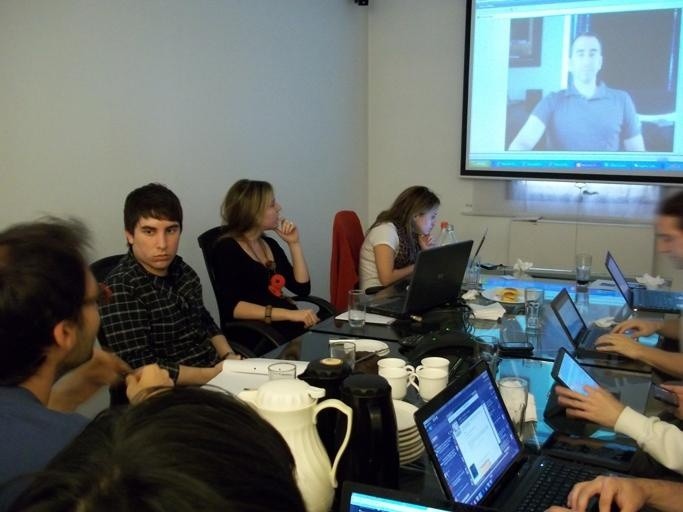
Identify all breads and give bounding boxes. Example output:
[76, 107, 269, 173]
[496, 287, 521, 302]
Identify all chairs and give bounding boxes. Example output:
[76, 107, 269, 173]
[88, 252, 256, 408]
[330, 209, 365, 313]
[198, 224, 340, 359]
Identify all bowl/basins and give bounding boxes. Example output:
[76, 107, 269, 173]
[481, 287, 540, 304]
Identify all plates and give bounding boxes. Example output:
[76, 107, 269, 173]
[321, 358, 341, 366]
[391, 399, 425, 466]
[343, 339, 389, 357]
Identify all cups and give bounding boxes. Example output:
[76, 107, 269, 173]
[524, 286, 545, 330]
[522, 330, 542, 368]
[409, 368, 449, 404]
[575, 283, 589, 312]
[330, 342, 356, 372]
[348, 290, 366, 328]
[465, 255, 481, 290]
[377, 358, 414, 380]
[416, 357, 450, 384]
[498, 377, 529, 440]
[475, 336, 499, 381]
[377, 368, 415, 400]
[268, 363, 296, 381]
[575, 253, 593, 282]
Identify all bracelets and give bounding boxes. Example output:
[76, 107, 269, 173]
[264, 304, 272, 326]
[221, 351, 236, 361]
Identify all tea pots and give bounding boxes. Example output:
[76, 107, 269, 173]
[334, 374, 401, 484]
[236, 378, 353, 512]
[297, 357, 365, 461]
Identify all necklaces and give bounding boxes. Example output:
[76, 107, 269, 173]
[244, 236, 273, 271]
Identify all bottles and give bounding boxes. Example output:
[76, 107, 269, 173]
[434, 222, 449, 246]
[440, 222, 458, 246]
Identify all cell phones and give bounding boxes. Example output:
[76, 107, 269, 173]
[480, 263, 496, 270]
[651, 382, 679, 408]
[499, 341, 534, 353]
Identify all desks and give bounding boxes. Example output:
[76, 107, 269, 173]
[202, 261, 672, 512]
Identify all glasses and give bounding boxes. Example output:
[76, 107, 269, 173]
[71, 279, 112, 310]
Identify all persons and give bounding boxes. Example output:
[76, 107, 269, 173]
[13, 382, 313, 511]
[96, 182, 245, 390]
[358, 186, 440, 308]
[594, 192, 683, 381]
[554, 382, 683, 473]
[1, 215, 175, 512]
[507, 32, 646, 152]
[544, 474, 683, 512]
[210, 179, 319, 355]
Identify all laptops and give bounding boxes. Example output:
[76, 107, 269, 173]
[339, 480, 498, 512]
[604, 250, 683, 315]
[550, 287, 638, 360]
[357, 240, 474, 319]
[413, 359, 638, 512]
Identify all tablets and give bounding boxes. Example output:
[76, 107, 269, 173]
[552, 348, 602, 397]
